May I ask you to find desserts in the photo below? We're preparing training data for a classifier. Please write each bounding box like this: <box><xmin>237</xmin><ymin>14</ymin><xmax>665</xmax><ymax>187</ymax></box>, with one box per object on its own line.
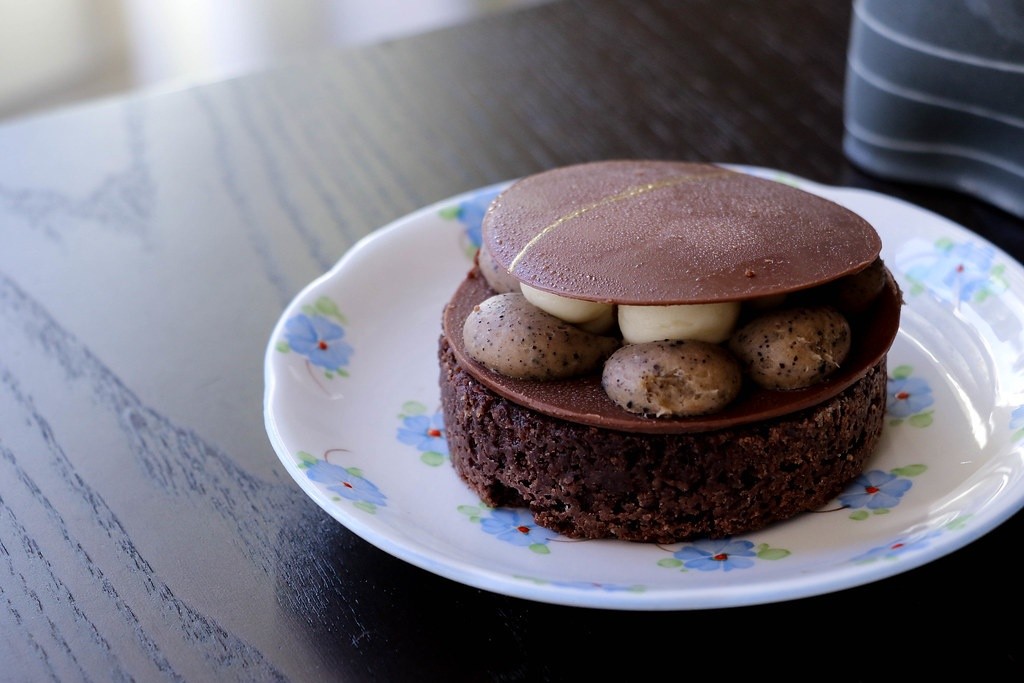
<box><xmin>437</xmin><ymin>160</ymin><xmax>904</xmax><ymax>540</ymax></box>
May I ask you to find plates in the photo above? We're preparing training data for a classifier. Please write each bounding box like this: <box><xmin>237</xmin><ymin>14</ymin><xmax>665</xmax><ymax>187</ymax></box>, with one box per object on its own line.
<box><xmin>264</xmin><ymin>163</ymin><xmax>1024</xmax><ymax>609</ymax></box>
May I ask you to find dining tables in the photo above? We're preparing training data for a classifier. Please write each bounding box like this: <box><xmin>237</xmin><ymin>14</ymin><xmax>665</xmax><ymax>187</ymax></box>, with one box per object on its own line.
<box><xmin>0</xmin><ymin>0</ymin><xmax>1024</xmax><ymax>683</ymax></box>
